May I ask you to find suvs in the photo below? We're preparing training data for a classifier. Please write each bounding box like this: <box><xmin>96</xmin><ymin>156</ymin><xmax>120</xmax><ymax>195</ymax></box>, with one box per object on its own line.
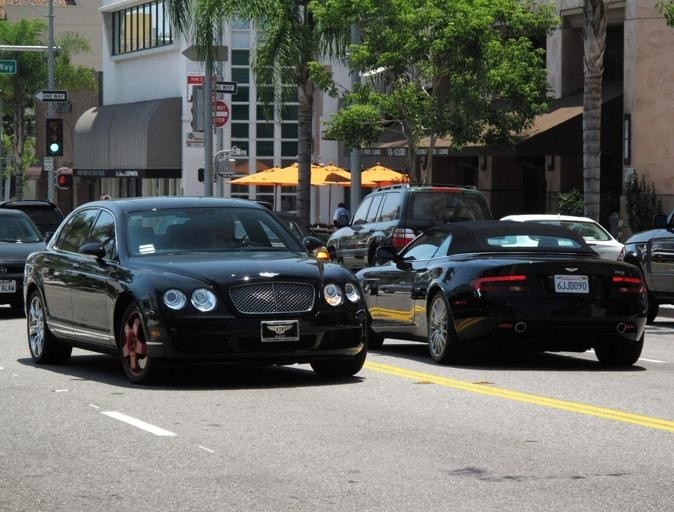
<box><xmin>325</xmin><ymin>184</ymin><xmax>494</xmax><ymax>272</ymax></box>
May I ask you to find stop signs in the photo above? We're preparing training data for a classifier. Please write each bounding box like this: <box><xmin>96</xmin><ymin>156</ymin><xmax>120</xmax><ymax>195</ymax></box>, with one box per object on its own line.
<box><xmin>212</xmin><ymin>100</ymin><xmax>229</xmax><ymax>128</ymax></box>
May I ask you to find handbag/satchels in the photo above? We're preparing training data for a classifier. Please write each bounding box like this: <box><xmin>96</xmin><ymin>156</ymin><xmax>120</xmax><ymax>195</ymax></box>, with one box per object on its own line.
<box><xmin>338</xmin><ymin>215</ymin><xmax>348</xmax><ymax>226</ymax></box>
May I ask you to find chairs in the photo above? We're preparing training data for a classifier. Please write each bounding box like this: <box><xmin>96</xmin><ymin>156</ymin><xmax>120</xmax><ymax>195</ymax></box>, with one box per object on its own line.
<box><xmin>166</xmin><ymin>223</ymin><xmax>199</xmax><ymax>250</ymax></box>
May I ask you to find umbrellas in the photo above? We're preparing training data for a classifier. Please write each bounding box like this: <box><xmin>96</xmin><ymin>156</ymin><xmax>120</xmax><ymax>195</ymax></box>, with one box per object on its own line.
<box><xmin>222</xmin><ymin>159</ymin><xmax>411</xmax><ymax>232</ymax></box>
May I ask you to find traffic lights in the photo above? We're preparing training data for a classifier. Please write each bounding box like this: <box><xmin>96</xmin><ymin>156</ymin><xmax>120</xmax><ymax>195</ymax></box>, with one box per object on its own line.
<box><xmin>57</xmin><ymin>174</ymin><xmax>73</xmax><ymax>188</ymax></box>
<box><xmin>46</xmin><ymin>118</ymin><xmax>63</xmax><ymax>156</ymax></box>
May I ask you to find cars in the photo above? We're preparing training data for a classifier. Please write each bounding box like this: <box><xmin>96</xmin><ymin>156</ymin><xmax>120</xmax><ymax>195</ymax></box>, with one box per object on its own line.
<box><xmin>353</xmin><ymin>219</ymin><xmax>649</xmax><ymax>368</ymax></box>
<box><xmin>498</xmin><ymin>213</ymin><xmax>627</xmax><ymax>262</ymax></box>
<box><xmin>1</xmin><ymin>199</ymin><xmax>65</xmax><ymax>241</ymax></box>
<box><xmin>0</xmin><ymin>208</ymin><xmax>54</xmax><ymax>317</ymax></box>
<box><xmin>624</xmin><ymin>210</ymin><xmax>674</xmax><ymax>325</ymax></box>
<box><xmin>23</xmin><ymin>195</ymin><xmax>371</xmax><ymax>384</ymax></box>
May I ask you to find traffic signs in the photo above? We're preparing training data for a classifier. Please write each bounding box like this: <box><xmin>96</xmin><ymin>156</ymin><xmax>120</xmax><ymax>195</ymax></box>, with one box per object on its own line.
<box><xmin>0</xmin><ymin>59</ymin><xmax>17</xmax><ymax>74</ymax></box>
<box><xmin>32</xmin><ymin>90</ymin><xmax>68</xmax><ymax>104</ymax></box>
<box><xmin>214</xmin><ymin>81</ymin><xmax>237</xmax><ymax>94</ymax></box>
